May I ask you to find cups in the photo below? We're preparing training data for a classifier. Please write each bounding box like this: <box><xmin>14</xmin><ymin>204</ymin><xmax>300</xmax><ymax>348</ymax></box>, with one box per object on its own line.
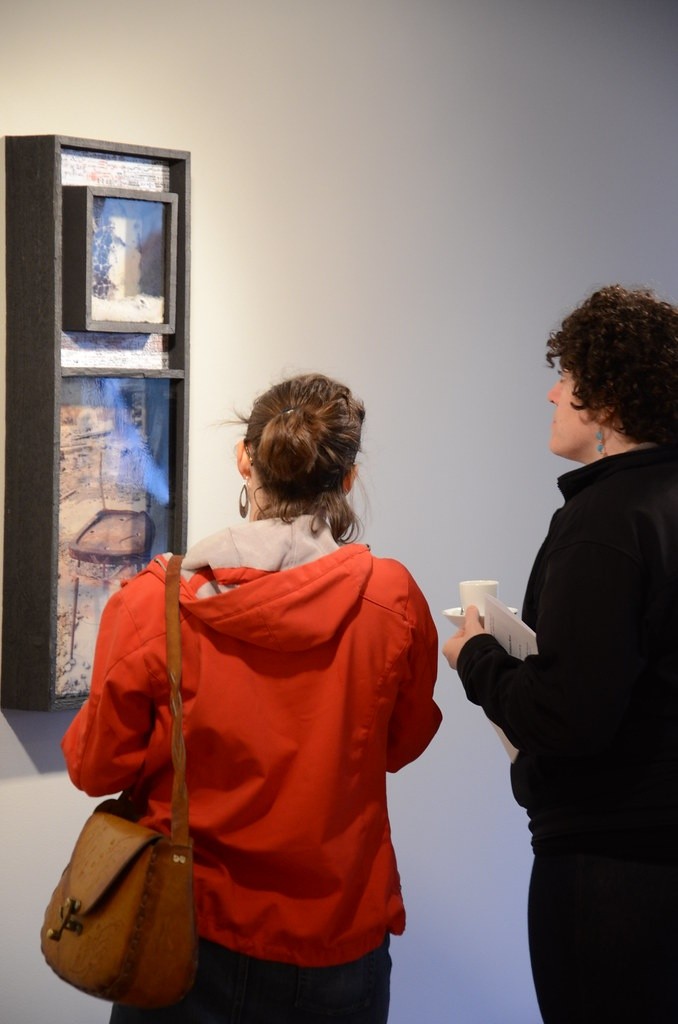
<box><xmin>459</xmin><ymin>580</ymin><xmax>499</xmax><ymax>617</ymax></box>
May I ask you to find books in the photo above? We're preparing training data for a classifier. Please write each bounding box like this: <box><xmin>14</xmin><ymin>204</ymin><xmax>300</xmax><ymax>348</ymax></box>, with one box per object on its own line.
<box><xmin>485</xmin><ymin>593</ymin><xmax>538</xmax><ymax>764</ymax></box>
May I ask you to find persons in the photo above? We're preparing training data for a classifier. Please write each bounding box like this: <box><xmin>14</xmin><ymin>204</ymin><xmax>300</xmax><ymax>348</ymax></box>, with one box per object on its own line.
<box><xmin>441</xmin><ymin>286</ymin><xmax>678</xmax><ymax>1023</ymax></box>
<box><xmin>60</xmin><ymin>373</ymin><xmax>443</xmax><ymax>1024</ymax></box>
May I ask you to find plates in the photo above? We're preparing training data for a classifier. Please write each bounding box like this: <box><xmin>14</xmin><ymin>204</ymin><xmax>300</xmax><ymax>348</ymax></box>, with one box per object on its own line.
<box><xmin>441</xmin><ymin>607</ymin><xmax>519</xmax><ymax>630</ymax></box>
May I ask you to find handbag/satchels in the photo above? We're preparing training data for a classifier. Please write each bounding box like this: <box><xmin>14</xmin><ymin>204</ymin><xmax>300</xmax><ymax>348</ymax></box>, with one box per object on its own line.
<box><xmin>40</xmin><ymin>798</ymin><xmax>197</xmax><ymax>1006</ymax></box>
